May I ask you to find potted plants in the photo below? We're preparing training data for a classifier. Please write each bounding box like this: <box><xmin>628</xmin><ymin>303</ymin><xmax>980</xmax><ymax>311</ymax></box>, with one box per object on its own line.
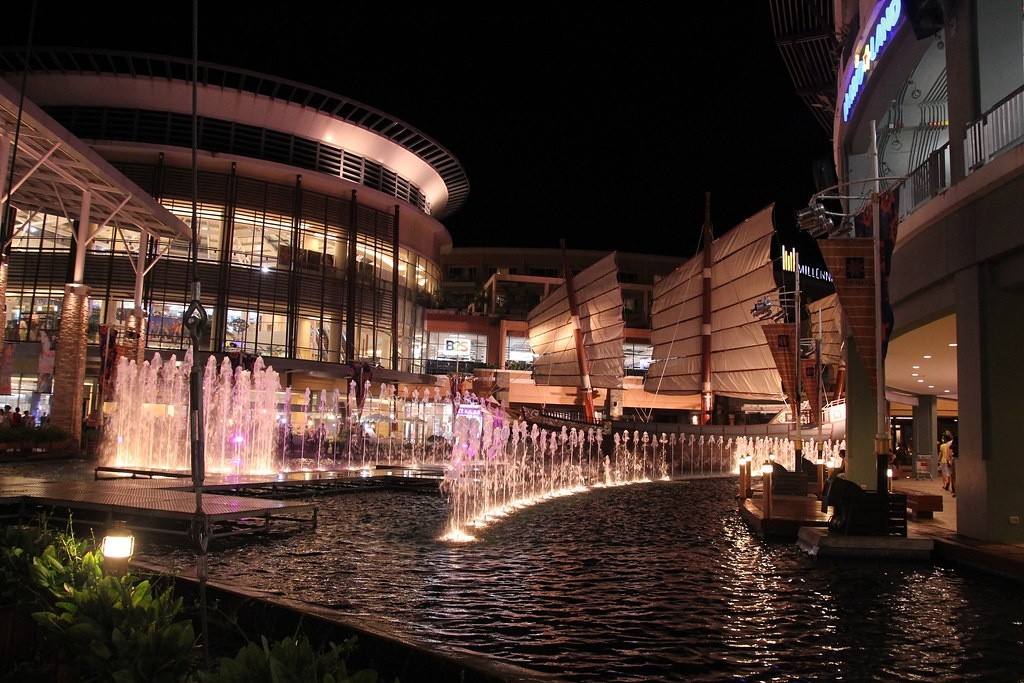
<box><xmin>0</xmin><ymin>423</ymin><xmax>72</xmax><ymax>451</ymax></box>
<box><xmin>83</xmin><ymin>429</ymin><xmax>97</xmax><ymax>449</ymax></box>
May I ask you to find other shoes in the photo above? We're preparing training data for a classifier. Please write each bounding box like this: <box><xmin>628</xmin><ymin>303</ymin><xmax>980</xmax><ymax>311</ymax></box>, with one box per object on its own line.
<box><xmin>943</xmin><ymin>482</ymin><xmax>949</xmax><ymax>490</ymax></box>
<box><xmin>951</xmin><ymin>490</ymin><xmax>955</xmax><ymax>493</ymax></box>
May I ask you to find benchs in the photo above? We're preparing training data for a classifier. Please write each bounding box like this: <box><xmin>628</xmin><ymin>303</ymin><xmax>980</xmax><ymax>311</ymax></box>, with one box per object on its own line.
<box><xmin>891</xmin><ymin>486</ymin><xmax>944</xmax><ymax>521</ymax></box>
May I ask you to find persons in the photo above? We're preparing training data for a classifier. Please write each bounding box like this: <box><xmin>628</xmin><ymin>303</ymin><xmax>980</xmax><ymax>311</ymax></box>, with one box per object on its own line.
<box><xmin>817</xmin><ymin>449</ymin><xmax>845</xmax><ymax>501</ymax></box>
<box><xmin>889</xmin><ymin>451</ymin><xmax>899</xmax><ymax>477</ymax></box>
<box><xmin>38</xmin><ymin>373</ymin><xmax>51</xmax><ymax>392</ymax></box>
<box><xmin>935</xmin><ymin>431</ymin><xmax>958</xmax><ymax>497</ymax></box>
<box><xmin>0</xmin><ymin>406</ymin><xmax>48</xmax><ymax>431</ymax></box>
